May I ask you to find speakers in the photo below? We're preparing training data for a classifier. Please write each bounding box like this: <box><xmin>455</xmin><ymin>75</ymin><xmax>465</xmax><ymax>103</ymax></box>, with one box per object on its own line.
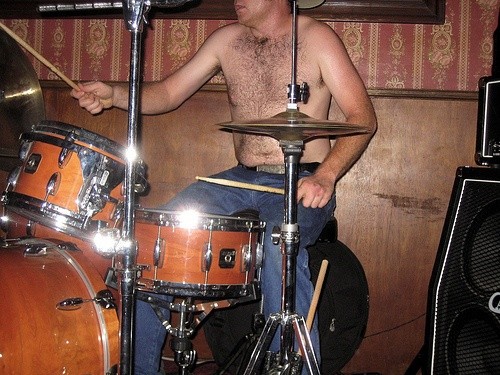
<box><xmin>422</xmin><ymin>167</ymin><xmax>500</xmax><ymax>374</ymax></box>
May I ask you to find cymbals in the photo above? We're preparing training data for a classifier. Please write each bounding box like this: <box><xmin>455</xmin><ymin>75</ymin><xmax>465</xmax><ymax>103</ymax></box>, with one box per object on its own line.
<box><xmin>215</xmin><ymin>111</ymin><xmax>372</xmax><ymax>140</ymax></box>
<box><xmin>0</xmin><ymin>31</ymin><xmax>46</xmax><ymax>170</ymax></box>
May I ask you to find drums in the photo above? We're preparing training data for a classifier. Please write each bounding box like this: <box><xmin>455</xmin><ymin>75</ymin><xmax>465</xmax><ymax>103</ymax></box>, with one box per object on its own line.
<box><xmin>104</xmin><ymin>205</ymin><xmax>267</xmax><ymax>297</ymax></box>
<box><xmin>0</xmin><ymin>236</ymin><xmax>122</xmax><ymax>374</ymax></box>
<box><xmin>2</xmin><ymin>120</ymin><xmax>149</xmax><ymax>258</ymax></box>
